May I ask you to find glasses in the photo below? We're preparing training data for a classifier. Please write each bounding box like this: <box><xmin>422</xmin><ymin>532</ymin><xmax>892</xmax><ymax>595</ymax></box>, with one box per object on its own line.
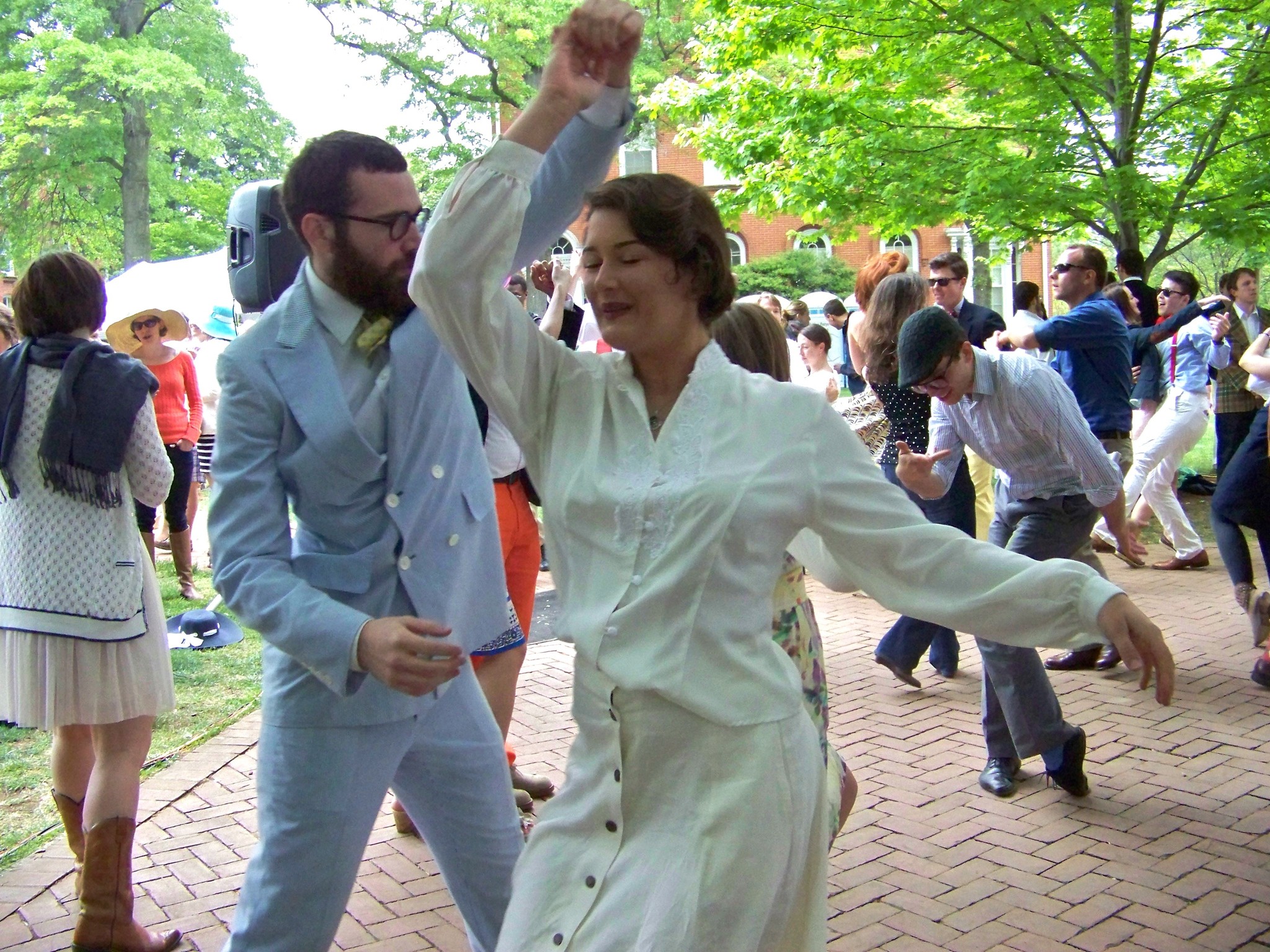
<box><xmin>132</xmin><ymin>319</ymin><xmax>159</xmax><ymax>331</ymax></box>
<box><xmin>1156</xmin><ymin>288</ymin><xmax>1185</xmax><ymax>297</ymax></box>
<box><xmin>339</xmin><ymin>209</ymin><xmax>430</xmax><ymax>241</ymax></box>
<box><xmin>928</xmin><ymin>277</ymin><xmax>961</xmax><ymax>287</ymax></box>
<box><xmin>1114</xmin><ymin>265</ymin><xmax>1119</xmax><ymax>271</ymax></box>
<box><xmin>1051</xmin><ymin>264</ymin><xmax>1088</xmax><ymax>273</ymax></box>
<box><xmin>911</xmin><ymin>348</ymin><xmax>956</xmax><ymax>394</ymax></box>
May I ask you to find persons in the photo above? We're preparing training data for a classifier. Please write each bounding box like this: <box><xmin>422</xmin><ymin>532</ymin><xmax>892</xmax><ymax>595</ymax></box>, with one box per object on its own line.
<box><xmin>408</xmin><ymin>11</ymin><xmax>1175</xmax><ymax>952</ymax></box>
<box><xmin>205</xmin><ymin>1</ymin><xmax>646</xmax><ymax>952</ymax></box>
<box><xmin>394</xmin><ymin>255</ymin><xmax>616</xmax><ymax>835</ymax></box>
<box><xmin>0</xmin><ymin>251</ymin><xmax>185</xmax><ymax>952</ymax></box>
<box><xmin>707</xmin><ymin>302</ymin><xmax>856</xmax><ymax>852</ymax></box>
<box><xmin>895</xmin><ymin>306</ymin><xmax>1153</xmax><ymax>798</ymax></box>
<box><xmin>0</xmin><ymin>300</ymin><xmax>243</xmax><ymax>601</ymax></box>
<box><xmin>740</xmin><ymin>244</ymin><xmax>1270</xmax><ymax>682</ymax></box>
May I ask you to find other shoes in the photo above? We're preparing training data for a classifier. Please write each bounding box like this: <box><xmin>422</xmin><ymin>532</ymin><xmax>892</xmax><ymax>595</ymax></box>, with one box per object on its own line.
<box><xmin>1251</xmin><ymin>648</ymin><xmax>1270</xmax><ymax>687</ymax></box>
<box><xmin>1089</xmin><ymin>530</ymin><xmax>1210</xmax><ymax>569</ymax></box>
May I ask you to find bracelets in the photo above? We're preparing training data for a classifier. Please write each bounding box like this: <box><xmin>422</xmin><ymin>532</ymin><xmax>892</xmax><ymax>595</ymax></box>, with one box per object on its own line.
<box><xmin>1262</xmin><ymin>333</ymin><xmax>1269</xmax><ymax>336</ymax></box>
<box><xmin>1212</xmin><ymin>338</ymin><xmax>1222</xmax><ymax>345</ymax></box>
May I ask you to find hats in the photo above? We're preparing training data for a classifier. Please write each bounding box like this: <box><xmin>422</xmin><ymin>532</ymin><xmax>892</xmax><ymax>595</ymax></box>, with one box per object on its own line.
<box><xmin>898</xmin><ymin>306</ymin><xmax>966</xmax><ymax>390</ymax></box>
<box><xmin>168</xmin><ymin>609</ymin><xmax>243</xmax><ymax>649</ymax></box>
<box><xmin>105</xmin><ymin>309</ymin><xmax>188</xmax><ymax>352</ymax></box>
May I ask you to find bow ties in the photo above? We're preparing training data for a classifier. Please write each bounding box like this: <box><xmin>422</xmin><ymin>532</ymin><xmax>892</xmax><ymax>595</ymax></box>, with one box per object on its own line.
<box><xmin>949</xmin><ymin>311</ymin><xmax>960</xmax><ymax>319</ymax></box>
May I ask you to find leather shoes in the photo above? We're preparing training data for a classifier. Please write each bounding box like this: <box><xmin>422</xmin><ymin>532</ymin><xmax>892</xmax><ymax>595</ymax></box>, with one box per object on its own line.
<box><xmin>978</xmin><ymin>757</ymin><xmax>1021</xmax><ymax>796</ymax></box>
<box><xmin>1042</xmin><ymin>728</ymin><xmax>1089</xmax><ymax>797</ymax></box>
<box><xmin>875</xmin><ymin>654</ymin><xmax>921</xmax><ymax>688</ymax></box>
<box><xmin>1045</xmin><ymin>647</ymin><xmax>1097</xmax><ymax>671</ymax></box>
<box><xmin>1096</xmin><ymin>645</ymin><xmax>1121</xmax><ymax>670</ymax></box>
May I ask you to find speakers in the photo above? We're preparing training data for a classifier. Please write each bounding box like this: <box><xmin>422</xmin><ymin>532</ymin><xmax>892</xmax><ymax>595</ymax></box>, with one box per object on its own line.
<box><xmin>226</xmin><ymin>178</ymin><xmax>305</xmax><ymax>314</ymax></box>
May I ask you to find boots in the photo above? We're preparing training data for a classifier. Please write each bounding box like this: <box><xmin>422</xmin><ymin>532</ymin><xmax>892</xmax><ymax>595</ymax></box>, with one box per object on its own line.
<box><xmin>170</xmin><ymin>525</ymin><xmax>204</xmax><ymax>600</ymax></box>
<box><xmin>51</xmin><ymin>789</ymin><xmax>84</xmax><ymax>898</ymax></box>
<box><xmin>72</xmin><ymin>816</ymin><xmax>182</xmax><ymax>952</ymax></box>
<box><xmin>1234</xmin><ymin>582</ymin><xmax>1270</xmax><ymax>647</ymax></box>
<box><xmin>141</xmin><ymin>532</ymin><xmax>155</xmax><ymax>569</ymax></box>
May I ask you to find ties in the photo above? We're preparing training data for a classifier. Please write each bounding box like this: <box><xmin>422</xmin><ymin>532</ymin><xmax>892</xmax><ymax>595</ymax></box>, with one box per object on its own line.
<box><xmin>356</xmin><ymin>316</ymin><xmax>394</xmax><ymax>356</ymax></box>
<box><xmin>1169</xmin><ymin>331</ymin><xmax>1178</xmax><ymax>383</ymax></box>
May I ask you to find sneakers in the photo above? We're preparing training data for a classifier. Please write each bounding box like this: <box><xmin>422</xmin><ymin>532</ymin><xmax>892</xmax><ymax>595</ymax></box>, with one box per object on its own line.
<box><xmin>156</xmin><ymin>538</ymin><xmax>193</xmax><ymax>552</ymax></box>
<box><xmin>394</xmin><ymin>787</ymin><xmax>533</xmax><ymax>834</ymax></box>
<box><xmin>507</xmin><ymin>755</ymin><xmax>554</xmax><ymax>797</ymax></box>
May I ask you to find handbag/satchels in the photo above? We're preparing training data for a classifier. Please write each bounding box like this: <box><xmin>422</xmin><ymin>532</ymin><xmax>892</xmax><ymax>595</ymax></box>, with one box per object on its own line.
<box><xmin>1181</xmin><ymin>475</ymin><xmax>1218</xmax><ymax>495</ymax></box>
